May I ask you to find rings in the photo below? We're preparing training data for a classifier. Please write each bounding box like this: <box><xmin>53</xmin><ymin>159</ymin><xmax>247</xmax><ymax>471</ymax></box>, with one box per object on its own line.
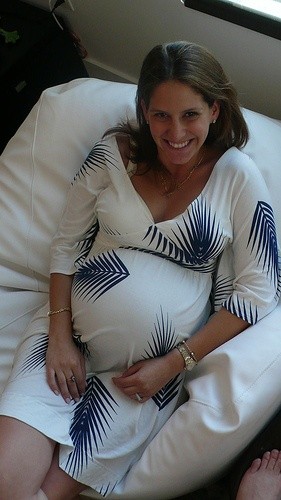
<box><xmin>67</xmin><ymin>376</ymin><xmax>76</xmax><ymax>382</ymax></box>
<box><xmin>134</xmin><ymin>393</ymin><xmax>143</xmax><ymax>402</ymax></box>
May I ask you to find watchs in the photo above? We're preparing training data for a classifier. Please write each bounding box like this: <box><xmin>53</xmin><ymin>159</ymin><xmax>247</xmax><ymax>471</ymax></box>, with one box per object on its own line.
<box><xmin>176</xmin><ymin>344</ymin><xmax>198</xmax><ymax>371</ymax></box>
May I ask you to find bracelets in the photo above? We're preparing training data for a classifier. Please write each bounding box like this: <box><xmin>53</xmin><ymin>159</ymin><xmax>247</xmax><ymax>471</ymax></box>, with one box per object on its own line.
<box><xmin>47</xmin><ymin>307</ymin><xmax>71</xmax><ymax>316</ymax></box>
<box><xmin>183</xmin><ymin>340</ymin><xmax>198</xmax><ymax>363</ymax></box>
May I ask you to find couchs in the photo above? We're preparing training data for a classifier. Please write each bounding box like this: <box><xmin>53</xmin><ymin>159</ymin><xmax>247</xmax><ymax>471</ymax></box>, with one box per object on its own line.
<box><xmin>0</xmin><ymin>78</ymin><xmax>280</xmax><ymax>499</ymax></box>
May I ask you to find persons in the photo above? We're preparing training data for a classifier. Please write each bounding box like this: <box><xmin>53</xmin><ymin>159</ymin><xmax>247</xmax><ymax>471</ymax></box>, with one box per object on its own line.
<box><xmin>0</xmin><ymin>42</ymin><xmax>281</xmax><ymax>500</ymax></box>
<box><xmin>237</xmin><ymin>449</ymin><xmax>281</xmax><ymax>500</ymax></box>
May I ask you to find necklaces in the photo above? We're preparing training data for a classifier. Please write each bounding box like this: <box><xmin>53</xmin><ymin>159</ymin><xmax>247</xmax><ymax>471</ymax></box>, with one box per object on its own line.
<box><xmin>160</xmin><ymin>150</ymin><xmax>205</xmax><ymax>199</ymax></box>
